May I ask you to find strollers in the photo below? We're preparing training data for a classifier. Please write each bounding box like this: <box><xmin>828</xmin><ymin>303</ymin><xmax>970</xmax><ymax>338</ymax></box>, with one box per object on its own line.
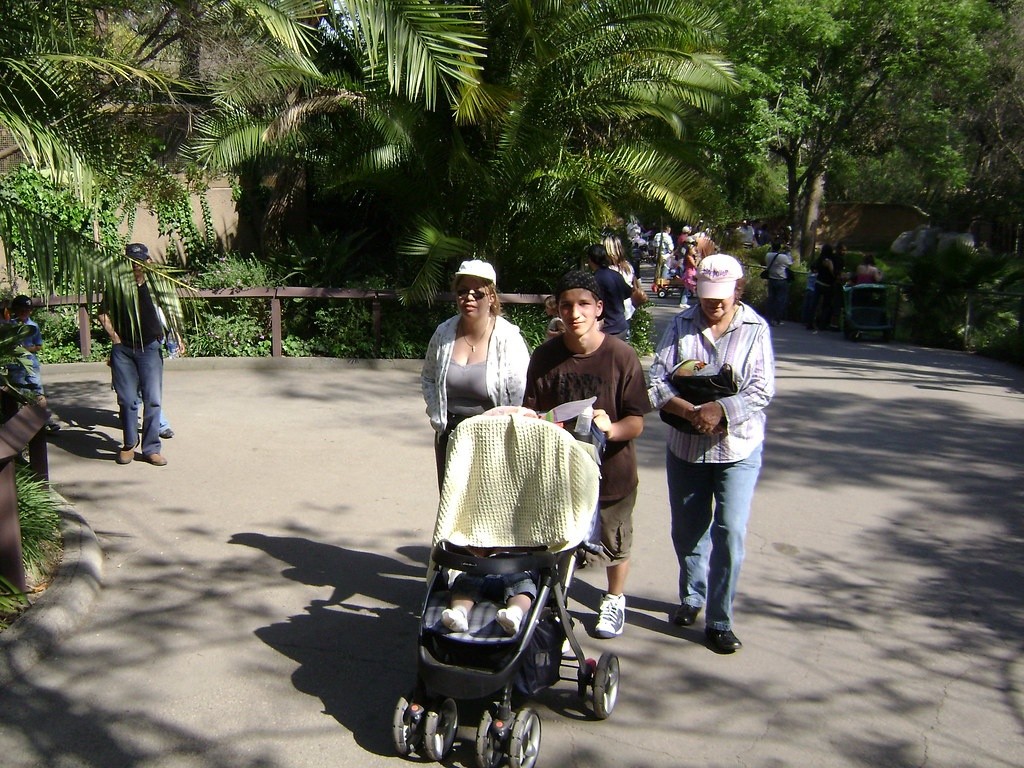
<box><xmin>392</xmin><ymin>412</ymin><xmax>621</xmax><ymax>768</ymax></box>
<box><xmin>843</xmin><ymin>282</ymin><xmax>894</xmax><ymax>341</ymax></box>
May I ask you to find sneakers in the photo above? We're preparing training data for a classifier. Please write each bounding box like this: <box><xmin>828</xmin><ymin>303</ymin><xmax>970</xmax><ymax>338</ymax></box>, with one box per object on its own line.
<box><xmin>594</xmin><ymin>592</ymin><xmax>626</xmax><ymax>638</ymax></box>
<box><xmin>44</xmin><ymin>421</ymin><xmax>60</xmax><ymax>431</ymax></box>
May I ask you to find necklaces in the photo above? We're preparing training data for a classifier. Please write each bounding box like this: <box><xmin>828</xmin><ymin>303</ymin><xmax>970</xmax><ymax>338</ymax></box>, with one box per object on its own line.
<box><xmin>461</xmin><ymin>318</ymin><xmax>491</xmax><ymax>352</ymax></box>
<box><xmin>128</xmin><ymin>274</ymin><xmax>157</xmax><ymax>294</ymax></box>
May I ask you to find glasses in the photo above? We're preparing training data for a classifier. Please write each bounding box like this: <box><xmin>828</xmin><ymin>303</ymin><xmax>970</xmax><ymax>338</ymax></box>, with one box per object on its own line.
<box><xmin>457</xmin><ymin>288</ymin><xmax>489</xmax><ymax>300</ymax></box>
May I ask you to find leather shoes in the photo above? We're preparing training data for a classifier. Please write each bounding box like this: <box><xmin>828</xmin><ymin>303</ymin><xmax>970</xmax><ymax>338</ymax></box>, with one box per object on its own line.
<box><xmin>673</xmin><ymin>601</ymin><xmax>701</xmax><ymax>625</ymax></box>
<box><xmin>705</xmin><ymin>625</ymin><xmax>742</xmax><ymax>649</ymax></box>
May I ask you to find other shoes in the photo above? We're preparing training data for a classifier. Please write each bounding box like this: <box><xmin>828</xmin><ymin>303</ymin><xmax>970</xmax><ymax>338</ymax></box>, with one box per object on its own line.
<box><xmin>768</xmin><ymin>319</ymin><xmax>785</xmax><ymax>325</ymax></box>
<box><xmin>441</xmin><ymin>609</ymin><xmax>469</xmax><ymax>632</ymax></box>
<box><xmin>161</xmin><ymin>429</ymin><xmax>174</xmax><ymax>438</ymax></box>
<box><xmin>495</xmin><ymin>608</ymin><xmax>520</xmax><ymax>634</ymax></box>
<box><xmin>116</xmin><ymin>434</ymin><xmax>140</xmax><ymax>463</ymax></box>
<box><xmin>679</xmin><ymin>303</ymin><xmax>690</xmax><ymax>308</ymax></box>
<box><xmin>143</xmin><ymin>451</ymin><xmax>167</xmax><ymax>466</ymax></box>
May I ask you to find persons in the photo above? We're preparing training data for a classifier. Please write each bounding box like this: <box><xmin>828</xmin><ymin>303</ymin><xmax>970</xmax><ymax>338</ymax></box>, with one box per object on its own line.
<box><xmin>649</xmin><ymin>254</ymin><xmax>776</xmax><ymax>651</ymax></box>
<box><xmin>765</xmin><ymin>237</ymin><xmax>882</xmax><ymax>331</ymax></box>
<box><xmin>544</xmin><ymin>294</ymin><xmax>568</xmax><ymax>348</ymax></box>
<box><xmin>652</xmin><ymin>225</ymin><xmax>715</xmax><ymax>309</ymax></box>
<box><xmin>98</xmin><ymin>243</ymin><xmax>185</xmax><ymax>466</ymax></box>
<box><xmin>734</xmin><ymin>218</ymin><xmax>767</xmax><ymax>250</ymax></box>
<box><xmin>521</xmin><ymin>270</ymin><xmax>652</xmax><ymax>640</ymax></box>
<box><xmin>441</xmin><ymin>546</ymin><xmax>539</xmax><ymax>636</ymax></box>
<box><xmin>0</xmin><ymin>295</ymin><xmax>47</xmax><ymax>409</ymax></box>
<box><xmin>421</xmin><ymin>260</ymin><xmax>532</xmax><ymax>498</ymax></box>
<box><xmin>587</xmin><ymin>234</ymin><xmax>636</xmax><ymax>342</ymax></box>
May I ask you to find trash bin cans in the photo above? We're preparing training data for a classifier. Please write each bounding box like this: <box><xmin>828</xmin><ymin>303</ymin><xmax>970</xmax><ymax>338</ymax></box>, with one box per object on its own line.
<box><xmin>0</xmin><ymin>386</ymin><xmax>53</xmax><ymax>593</ymax></box>
<box><xmin>841</xmin><ymin>283</ymin><xmax>894</xmax><ymax>341</ymax></box>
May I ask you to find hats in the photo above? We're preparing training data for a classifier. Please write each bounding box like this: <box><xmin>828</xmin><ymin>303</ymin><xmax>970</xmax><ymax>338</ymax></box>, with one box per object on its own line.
<box><xmin>12</xmin><ymin>294</ymin><xmax>33</xmax><ymax>310</ymax></box>
<box><xmin>125</xmin><ymin>243</ymin><xmax>148</xmax><ymax>262</ymax></box>
<box><xmin>695</xmin><ymin>253</ymin><xmax>744</xmax><ymax>299</ymax></box>
<box><xmin>682</xmin><ymin>226</ymin><xmax>692</xmax><ymax>234</ymax></box>
<box><xmin>455</xmin><ymin>260</ymin><xmax>497</xmax><ymax>288</ymax></box>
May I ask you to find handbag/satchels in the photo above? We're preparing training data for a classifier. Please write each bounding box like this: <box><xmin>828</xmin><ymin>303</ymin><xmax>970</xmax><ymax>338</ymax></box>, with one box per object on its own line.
<box><xmin>660</xmin><ymin>358</ymin><xmax>737</xmax><ymax>436</ymax></box>
<box><xmin>760</xmin><ymin>270</ymin><xmax>771</xmax><ymax>280</ymax></box>
<box><xmin>632</xmin><ymin>280</ymin><xmax>649</xmax><ymax>306</ymax></box>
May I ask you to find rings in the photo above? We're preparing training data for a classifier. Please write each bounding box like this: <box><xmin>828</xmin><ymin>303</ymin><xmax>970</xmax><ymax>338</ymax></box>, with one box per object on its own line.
<box><xmin>696</xmin><ymin>424</ymin><xmax>702</xmax><ymax>429</ymax></box>
<box><xmin>693</xmin><ymin>405</ymin><xmax>702</xmax><ymax>411</ymax></box>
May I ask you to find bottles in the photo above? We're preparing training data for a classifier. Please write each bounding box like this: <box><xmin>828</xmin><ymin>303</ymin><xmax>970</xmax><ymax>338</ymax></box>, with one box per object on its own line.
<box><xmin>167</xmin><ymin>341</ymin><xmax>179</xmax><ymax>360</ymax></box>
<box><xmin>574</xmin><ymin>404</ymin><xmax>593</xmax><ymax>435</ymax></box>
